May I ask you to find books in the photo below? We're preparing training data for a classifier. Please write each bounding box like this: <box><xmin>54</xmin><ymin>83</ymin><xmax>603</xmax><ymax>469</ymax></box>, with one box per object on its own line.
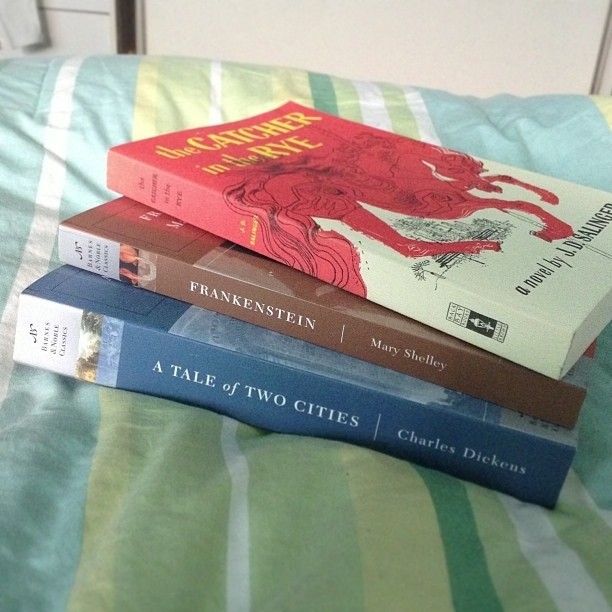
<box><xmin>56</xmin><ymin>194</ymin><xmax>596</xmax><ymax>428</ymax></box>
<box><xmin>14</xmin><ymin>265</ymin><xmax>578</xmax><ymax>511</ymax></box>
<box><xmin>106</xmin><ymin>101</ymin><xmax>611</xmax><ymax>380</ymax></box>
